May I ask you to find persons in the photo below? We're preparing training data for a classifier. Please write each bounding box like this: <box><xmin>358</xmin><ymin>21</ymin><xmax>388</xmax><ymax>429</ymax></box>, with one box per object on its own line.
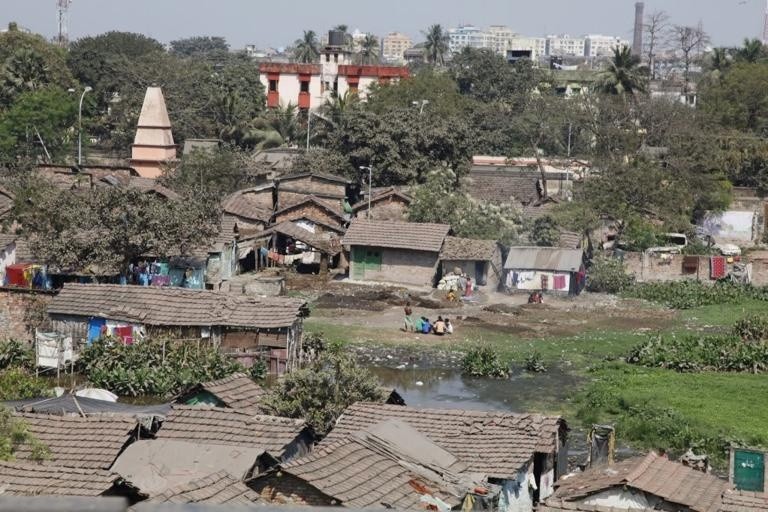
<box><xmin>416</xmin><ymin>314</ymin><xmax>454</xmax><ymax>335</ymax></box>
<box><xmin>529</xmin><ymin>291</ymin><xmax>544</xmax><ymax>304</ymax></box>
<box><xmin>288</xmin><ymin>240</ymin><xmax>295</xmax><ymax>255</ymax></box>
<box><xmin>657</xmin><ymin>446</ymin><xmax>669</xmax><ymax>459</ymax></box>
<box><xmin>465</xmin><ymin>278</ymin><xmax>472</xmax><ymax>297</ymax></box>
<box><xmin>403</xmin><ymin>301</ymin><xmax>414</xmax><ymax>332</ymax></box>
<box><xmin>447</xmin><ymin>289</ymin><xmax>456</xmax><ymax>301</ymax></box>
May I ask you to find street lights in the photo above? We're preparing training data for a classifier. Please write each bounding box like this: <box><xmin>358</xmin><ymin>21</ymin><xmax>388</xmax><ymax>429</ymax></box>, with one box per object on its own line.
<box><xmin>419</xmin><ymin>99</ymin><xmax>431</xmax><ymax>116</ymax></box>
<box><xmin>359</xmin><ymin>163</ymin><xmax>372</xmax><ymax>220</ymax></box>
<box><xmin>77</xmin><ymin>84</ymin><xmax>93</xmax><ymax>164</ymax></box>
<box><xmin>67</xmin><ymin>88</ymin><xmax>76</xmax><ymax>98</ymax></box>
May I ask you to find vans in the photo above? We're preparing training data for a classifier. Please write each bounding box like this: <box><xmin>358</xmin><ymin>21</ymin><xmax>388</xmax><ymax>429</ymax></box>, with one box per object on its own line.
<box><xmin>650</xmin><ymin>232</ymin><xmax>688</xmax><ymax>256</ymax></box>
